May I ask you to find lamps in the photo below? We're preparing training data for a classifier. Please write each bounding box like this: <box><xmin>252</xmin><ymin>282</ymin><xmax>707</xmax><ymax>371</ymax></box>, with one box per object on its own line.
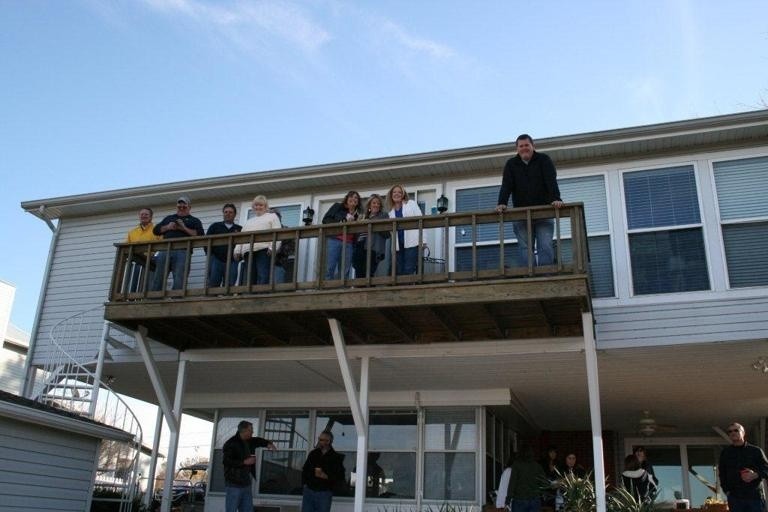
<box><xmin>433</xmin><ymin>193</ymin><xmax>449</xmax><ymax>214</ymax></box>
<box><xmin>637</xmin><ymin>426</ymin><xmax>655</xmax><ymax>437</ymax></box>
<box><xmin>301</xmin><ymin>205</ymin><xmax>315</xmax><ymax>226</ymax></box>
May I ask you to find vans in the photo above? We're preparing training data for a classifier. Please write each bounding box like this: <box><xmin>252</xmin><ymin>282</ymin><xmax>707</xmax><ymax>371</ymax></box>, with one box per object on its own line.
<box><xmin>155</xmin><ymin>462</ymin><xmax>209</xmax><ymax>505</ymax></box>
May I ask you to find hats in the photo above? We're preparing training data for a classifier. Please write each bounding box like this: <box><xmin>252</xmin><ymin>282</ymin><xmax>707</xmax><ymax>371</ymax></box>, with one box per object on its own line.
<box><xmin>177</xmin><ymin>196</ymin><xmax>190</xmax><ymax>205</ymax></box>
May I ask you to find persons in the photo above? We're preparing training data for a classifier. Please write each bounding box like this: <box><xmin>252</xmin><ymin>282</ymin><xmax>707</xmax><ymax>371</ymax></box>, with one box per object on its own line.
<box><xmin>204</xmin><ymin>204</ymin><xmax>243</xmax><ymax>297</ymax></box>
<box><xmin>222</xmin><ymin>422</ymin><xmax>276</xmax><ymax>512</ymax></box>
<box><xmin>321</xmin><ymin>190</ymin><xmax>361</xmax><ymax>290</ymax></box>
<box><xmin>301</xmin><ymin>429</ymin><xmax>347</xmax><ymax>512</ymax></box>
<box><xmin>351</xmin><ymin>194</ymin><xmax>392</xmax><ymax>288</ymax></box>
<box><xmin>718</xmin><ymin>421</ymin><xmax>767</xmax><ymax>511</ymax></box>
<box><xmin>494</xmin><ymin>134</ymin><xmax>564</xmax><ymax>278</ymax></box>
<box><xmin>385</xmin><ymin>184</ymin><xmax>428</xmax><ymax>286</ymax></box>
<box><xmin>267</xmin><ymin>208</ymin><xmax>293</xmax><ymax>283</ymax></box>
<box><xmin>233</xmin><ymin>195</ymin><xmax>281</xmax><ymax>294</ymax></box>
<box><xmin>490</xmin><ymin>443</ymin><xmax>660</xmax><ymax>510</ymax></box>
<box><xmin>150</xmin><ymin>196</ymin><xmax>205</xmax><ymax>300</ymax></box>
<box><xmin>123</xmin><ymin>207</ymin><xmax>161</xmax><ymax>302</ymax></box>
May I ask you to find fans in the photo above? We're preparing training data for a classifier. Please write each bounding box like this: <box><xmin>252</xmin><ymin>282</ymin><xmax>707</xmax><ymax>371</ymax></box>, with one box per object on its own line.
<box><xmin>611</xmin><ymin>408</ymin><xmax>676</xmax><ymax>428</ymax></box>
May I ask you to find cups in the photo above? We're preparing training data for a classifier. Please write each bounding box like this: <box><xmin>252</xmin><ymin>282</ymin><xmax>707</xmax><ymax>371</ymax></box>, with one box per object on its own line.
<box><xmin>315</xmin><ymin>467</ymin><xmax>321</xmax><ymax>476</ymax></box>
<box><xmin>251</xmin><ymin>454</ymin><xmax>255</xmax><ymax>457</ymax></box>
<box><xmin>740</xmin><ymin>470</ymin><xmax>751</xmax><ymax>482</ymax></box>
<box><xmin>346</xmin><ymin>214</ymin><xmax>354</xmax><ymax>222</ymax></box>
